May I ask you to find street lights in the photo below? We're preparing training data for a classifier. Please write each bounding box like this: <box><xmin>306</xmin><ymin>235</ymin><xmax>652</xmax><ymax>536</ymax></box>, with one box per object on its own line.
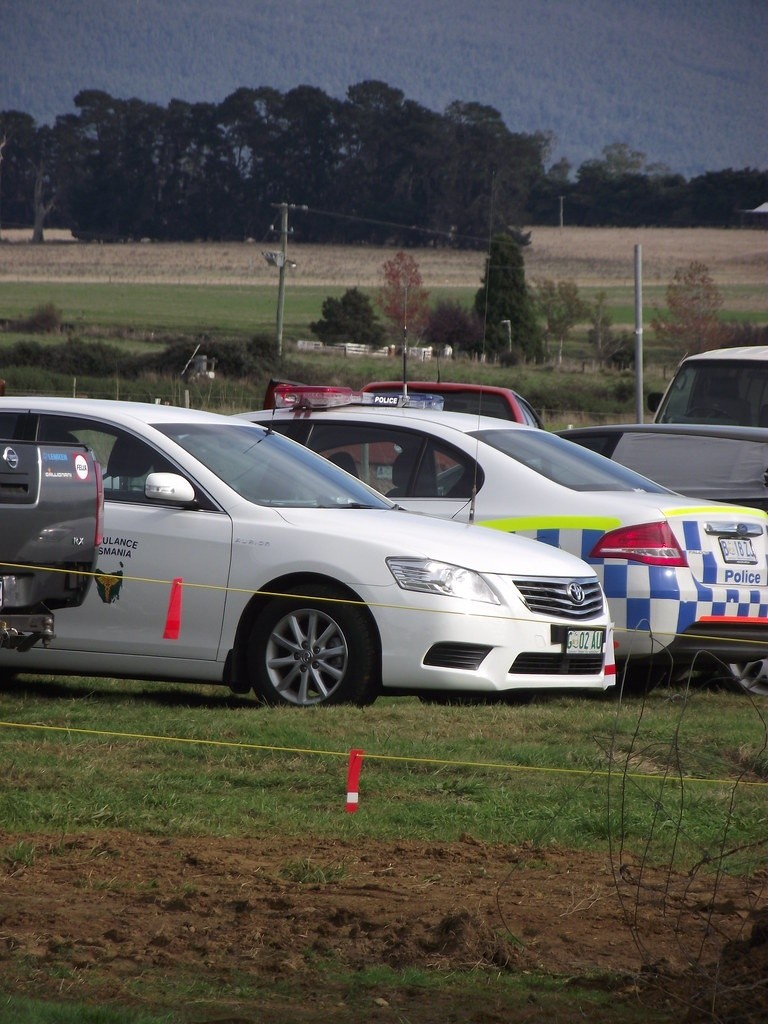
<box><xmin>501</xmin><ymin>319</ymin><xmax>512</xmax><ymax>353</ymax></box>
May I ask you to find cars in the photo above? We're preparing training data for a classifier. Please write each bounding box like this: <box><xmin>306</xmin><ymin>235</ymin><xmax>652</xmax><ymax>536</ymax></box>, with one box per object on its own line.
<box><xmin>0</xmin><ymin>395</ymin><xmax>619</xmax><ymax>712</ymax></box>
<box><xmin>537</xmin><ymin>346</ymin><xmax>768</xmax><ymax>517</ymax></box>
<box><xmin>262</xmin><ymin>380</ymin><xmax>546</xmax><ymax>497</ymax></box>
<box><xmin>102</xmin><ymin>385</ymin><xmax>768</xmax><ymax>700</ymax></box>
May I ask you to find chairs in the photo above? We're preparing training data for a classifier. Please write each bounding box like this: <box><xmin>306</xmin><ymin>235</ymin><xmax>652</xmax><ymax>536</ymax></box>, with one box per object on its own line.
<box><xmin>385</xmin><ymin>450</ymin><xmax>415</xmax><ymax>498</ymax></box>
<box><xmin>102</xmin><ymin>435</ymin><xmax>153</xmax><ymax>492</ymax></box>
<box><xmin>688</xmin><ymin>376</ymin><xmax>752</xmax><ymax>425</ymax></box>
<box><xmin>326</xmin><ymin>451</ymin><xmax>359</xmax><ymax>481</ymax></box>
